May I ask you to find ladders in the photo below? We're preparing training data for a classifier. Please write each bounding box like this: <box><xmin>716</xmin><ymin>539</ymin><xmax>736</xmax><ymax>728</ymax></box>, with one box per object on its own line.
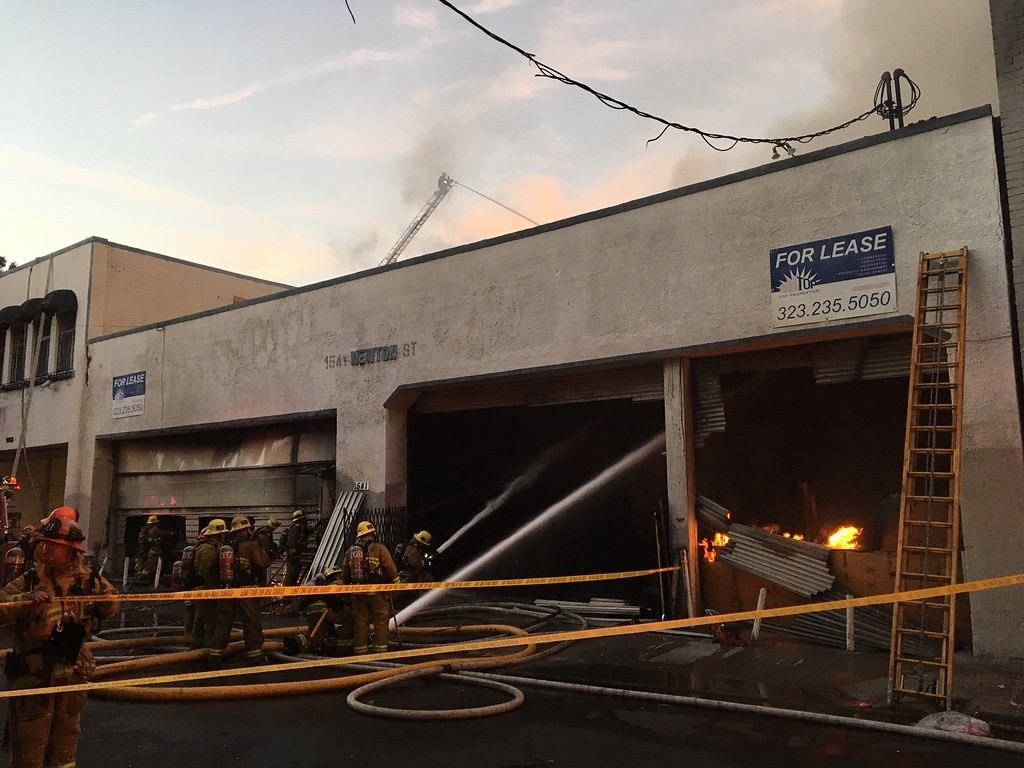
<box><xmin>887</xmin><ymin>244</ymin><xmax>971</xmax><ymax>713</ymax></box>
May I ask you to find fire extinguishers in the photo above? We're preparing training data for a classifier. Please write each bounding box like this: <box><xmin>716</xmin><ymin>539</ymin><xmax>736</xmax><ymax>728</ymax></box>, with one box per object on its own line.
<box><xmin>350</xmin><ymin>545</ymin><xmax>365</xmax><ymax>581</ymax></box>
<box><xmin>4</xmin><ymin>547</ymin><xmax>24</xmax><ymax>590</ymax></box>
<box><xmin>392</xmin><ymin>544</ymin><xmax>405</xmax><ymax>563</ymax></box>
<box><xmin>219</xmin><ymin>546</ymin><xmax>234</xmax><ymax>587</ymax></box>
<box><xmin>178</xmin><ymin>546</ymin><xmax>196</xmax><ymax>586</ymax></box>
<box><xmin>171</xmin><ymin>561</ymin><xmax>183</xmax><ymax>593</ymax></box>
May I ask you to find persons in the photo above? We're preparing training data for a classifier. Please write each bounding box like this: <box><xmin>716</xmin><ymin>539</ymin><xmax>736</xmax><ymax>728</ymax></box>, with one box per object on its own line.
<box><xmin>281</xmin><ymin>565</ymin><xmax>356</xmax><ymax>657</ymax></box>
<box><xmin>133</xmin><ymin>515</ymin><xmax>181</xmax><ymax>581</ymax></box>
<box><xmin>283</xmin><ymin>509</ymin><xmax>323</xmax><ymax>601</ymax></box>
<box><xmin>184</xmin><ymin>515</ymin><xmax>282</xmax><ymax>670</ymax></box>
<box><xmin>393</xmin><ymin>530</ymin><xmax>431</xmax><ymax>626</ymax></box>
<box><xmin>0</xmin><ymin>507</ymin><xmax>124</xmax><ymax>768</ymax></box>
<box><xmin>341</xmin><ymin>521</ymin><xmax>402</xmax><ymax>660</ymax></box>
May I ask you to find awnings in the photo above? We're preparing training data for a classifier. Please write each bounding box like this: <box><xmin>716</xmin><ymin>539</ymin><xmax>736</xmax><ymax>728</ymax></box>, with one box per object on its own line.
<box><xmin>16</xmin><ymin>298</ymin><xmax>42</xmax><ymax>323</ymax></box>
<box><xmin>0</xmin><ymin>305</ymin><xmax>26</xmax><ymax>324</ymax></box>
<box><xmin>37</xmin><ymin>290</ymin><xmax>78</xmax><ymax>313</ymax></box>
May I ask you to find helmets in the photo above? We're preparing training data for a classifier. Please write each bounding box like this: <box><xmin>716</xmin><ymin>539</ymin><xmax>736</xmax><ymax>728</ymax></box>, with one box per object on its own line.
<box><xmin>146</xmin><ymin>516</ymin><xmax>159</xmax><ymax>524</ymax></box>
<box><xmin>203</xmin><ymin>519</ymin><xmax>229</xmax><ymax>535</ymax></box>
<box><xmin>324</xmin><ymin>566</ymin><xmax>343</xmax><ymax>576</ymax></box>
<box><xmin>36</xmin><ymin>517</ymin><xmax>88</xmax><ymax>553</ymax></box>
<box><xmin>266</xmin><ymin>519</ymin><xmax>279</xmax><ymax>529</ymax></box>
<box><xmin>41</xmin><ymin>507</ymin><xmax>78</xmax><ymax>525</ymax></box>
<box><xmin>292</xmin><ymin>510</ymin><xmax>305</xmax><ymax>521</ymax></box>
<box><xmin>414</xmin><ymin>531</ymin><xmax>431</xmax><ymax>545</ymax></box>
<box><xmin>355</xmin><ymin>521</ymin><xmax>376</xmax><ymax>538</ymax></box>
<box><xmin>229</xmin><ymin>517</ymin><xmax>251</xmax><ymax>533</ymax></box>
